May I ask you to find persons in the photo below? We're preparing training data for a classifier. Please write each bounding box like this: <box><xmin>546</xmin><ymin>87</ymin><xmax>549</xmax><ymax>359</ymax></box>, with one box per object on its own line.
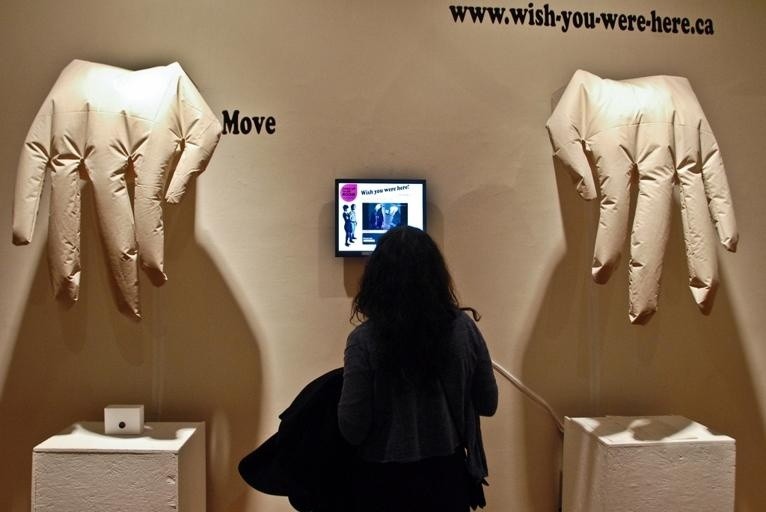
<box><xmin>338</xmin><ymin>225</ymin><xmax>498</xmax><ymax>512</ymax></box>
<box><xmin>342</xmin><ymin>204</ymin><xmax>357</xmax><ymax>246</ymax></box>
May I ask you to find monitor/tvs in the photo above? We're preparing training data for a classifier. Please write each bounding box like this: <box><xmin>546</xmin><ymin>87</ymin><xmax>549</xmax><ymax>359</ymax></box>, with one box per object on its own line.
<box><xmin>334</xmin><ymin>177</ymin><xmax>428</xmax><ymax>258</ymax></box>
